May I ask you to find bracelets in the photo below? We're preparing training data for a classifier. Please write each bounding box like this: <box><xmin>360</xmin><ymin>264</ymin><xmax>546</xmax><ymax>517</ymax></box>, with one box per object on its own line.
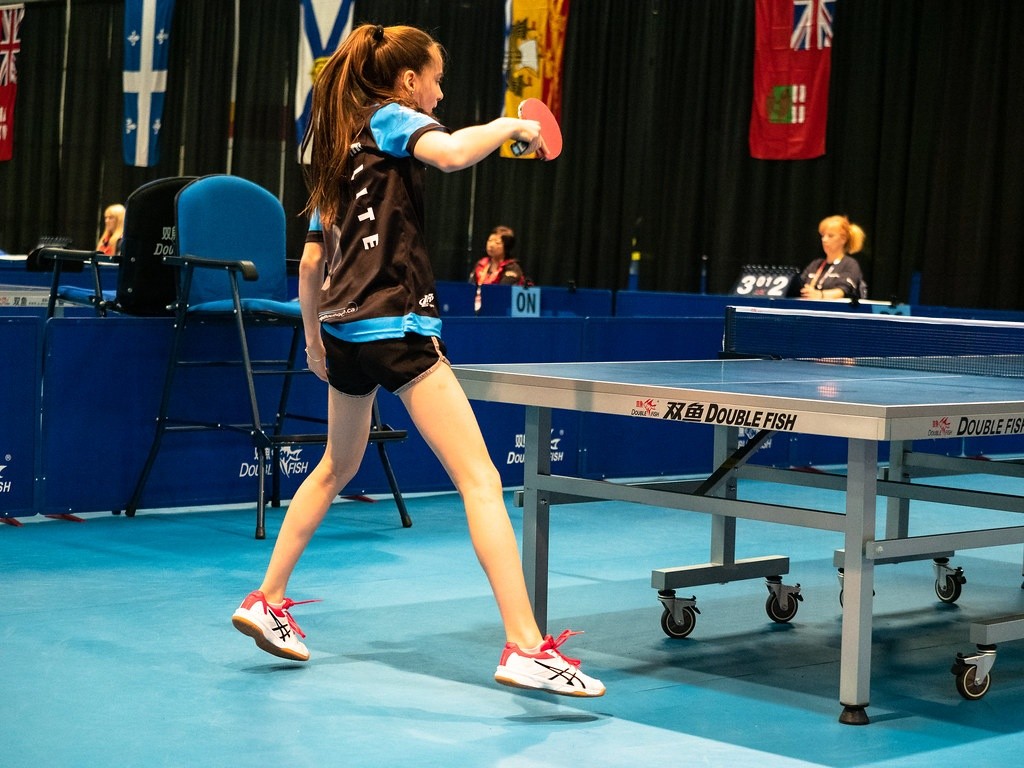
<box><xmin>819</xmin><ymin>289</ymin><xmax>825</xmax><ymax>299</ymax></box>
<box><xmin>304</xmin><ymin>346</ymin><xmax>327</xmax><ymax>361</ymax></box>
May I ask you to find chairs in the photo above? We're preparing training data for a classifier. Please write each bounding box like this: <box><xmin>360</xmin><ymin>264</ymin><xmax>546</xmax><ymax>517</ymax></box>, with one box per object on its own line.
<box><xmin>21</xmin><ymin>176</ymin><xmax>200</xmax><ymax>317</ymax></box>
<box><xmin>129</xmin><ymin>172</ymin><xmax>412</xmax><ymax>540</ymax></box>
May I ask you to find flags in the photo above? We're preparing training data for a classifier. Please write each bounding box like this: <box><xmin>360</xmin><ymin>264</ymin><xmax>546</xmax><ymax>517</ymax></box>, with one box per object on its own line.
<box><xmin>499</xmin><ymin>0</ymin><xmax>570</xmax><ymax>159</ymax></box>
<box><xmin>748</xmin><ymin>0</ymin><xmax>833</xmax><ymax>159</ymax></box>
<box><xmin>296</xmin><ymin>0</ymin><xmax>354</xmax><ymax>165</ymax></box>
<box><xmin>122</xmin><ymin>0</ymin><xmax>173</xmax><ymax>167</ymax></box>
<box><xmin>0</xmin><ymin>2</ymin><xmax>26</xmax><ymax>160</ymax></box>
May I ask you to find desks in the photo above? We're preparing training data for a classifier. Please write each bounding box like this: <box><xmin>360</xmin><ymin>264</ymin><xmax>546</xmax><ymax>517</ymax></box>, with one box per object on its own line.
<box><xmin>451</xmin><ymin>306</ymin><xmax>1024</xmax><ymax>727</ymax></box>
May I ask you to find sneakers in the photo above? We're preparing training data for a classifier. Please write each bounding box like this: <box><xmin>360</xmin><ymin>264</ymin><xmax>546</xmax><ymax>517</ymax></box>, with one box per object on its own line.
<box><xmin>232</xmin><ymin>590</ymin><xmax>311</xmax><ymax>662</ymax></box>
<box><xmin>494</xmin><ymin>628</ymin><xmax>607</xmax><ymax>698</ymax></box>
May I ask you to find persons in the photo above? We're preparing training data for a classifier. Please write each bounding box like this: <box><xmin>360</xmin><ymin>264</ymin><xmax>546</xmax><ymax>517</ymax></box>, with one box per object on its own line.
<box><xmin>95</xmin><ymin>202</ymin><xmax>126</xmax><ymax>255</ymax></box>
<box><xmin>231</xmin><ymin>22</ymin><xmax>606</xmax><ymax>698</ymax></box>
<box><xmin>467</xmin><ymin>225</ymin><xmax>527</xmax><ymax>287</ymax></box>
<box><xmin>800</xmin><ymin>215</ymin><xmax>863</xmax><ymax>301</ymax></box>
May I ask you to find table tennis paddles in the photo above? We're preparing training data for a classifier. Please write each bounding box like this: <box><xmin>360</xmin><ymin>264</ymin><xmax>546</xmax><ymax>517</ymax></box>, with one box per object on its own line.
<box><xmin>510</xmin><ymin>96</ymin><xmax>563</xmax><ymax>162</ymax></box>
